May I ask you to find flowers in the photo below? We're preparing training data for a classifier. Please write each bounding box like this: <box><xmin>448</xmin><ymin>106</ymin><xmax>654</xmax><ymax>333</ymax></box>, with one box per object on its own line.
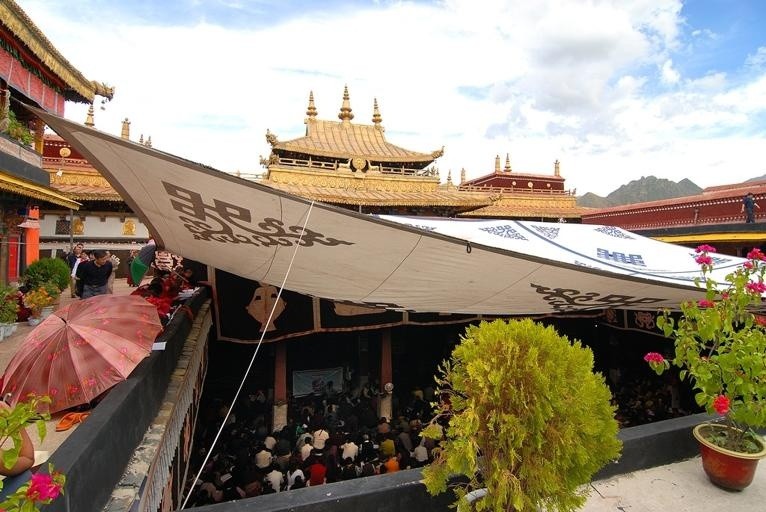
<box><xmin>645</xmin><ymin>243</ymin><xmax>766</xmax><ymax>446</ymax></box>
<box><xmin>26</xmin><ymin>286</ymin><xmax>52</xmax><ymax>316</ymax></box>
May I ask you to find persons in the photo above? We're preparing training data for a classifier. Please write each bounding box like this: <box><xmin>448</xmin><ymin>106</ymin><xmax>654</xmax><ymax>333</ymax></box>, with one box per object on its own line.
<box><xmin>131</xmin><ymin>262</ymin><xmax>211</xmax><ymax>328</ymax></box>
<box><xmin>741</xmin><ymin>192</ymin><xmax>761</xmax><ymax>223</ymax></box>
<box><xmin>601</xmin><ymin>370</ymin><xmax>699</xmax><ymax>429</ymax></box>
<box><xmin>0</xmin><ymin>400</ymin><xmax>35</xmax><ymax>478</ymax></box>
<box><xmin>125</xmin><ymin>250</ymin><xmax>138</xmax><ymax>287</ymax></box>
<box><xmin>61</xmin><ymin>244</ymin><xmax>120</xmax><ymax>301</ymax></box>
<box><xmin>182</xmin><ymin>372</ymin><xmax>452</xmax><ymax>508</ymax></box>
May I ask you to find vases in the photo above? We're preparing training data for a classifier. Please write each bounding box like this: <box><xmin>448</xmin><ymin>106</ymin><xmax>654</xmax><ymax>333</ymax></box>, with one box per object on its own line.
<box><xmin>28</xmin><ymin>306</ymin><xmax>54</xmax><ymax>326</ymax></box>
<box><xmin>693</xmin><ymin>422</ymin><xmax>766</xmax><ymax>492</ymax></box>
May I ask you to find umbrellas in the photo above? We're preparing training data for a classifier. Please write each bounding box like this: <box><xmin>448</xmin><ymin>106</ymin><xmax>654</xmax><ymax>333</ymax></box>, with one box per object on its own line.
<box><xmin>0</xmin><ymin>291</ymin><xmax>166</xmax><ymax>420</ymax></box>
<box><xmin>130</xmin><ymin>243</ymin><xmax>158</xmax><ymax>288</ymax></box>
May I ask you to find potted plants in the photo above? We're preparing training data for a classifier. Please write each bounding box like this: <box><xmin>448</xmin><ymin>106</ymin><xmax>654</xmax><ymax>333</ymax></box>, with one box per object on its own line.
<box><xmin>418</xmin><ymin>318</ymin><xmax>623</xmax><ymax>512</ymax></box>
<box><xmin>0</xmin><ymin>300</ymin><xmax>18</xmax><ymax>342</ymax></box>
